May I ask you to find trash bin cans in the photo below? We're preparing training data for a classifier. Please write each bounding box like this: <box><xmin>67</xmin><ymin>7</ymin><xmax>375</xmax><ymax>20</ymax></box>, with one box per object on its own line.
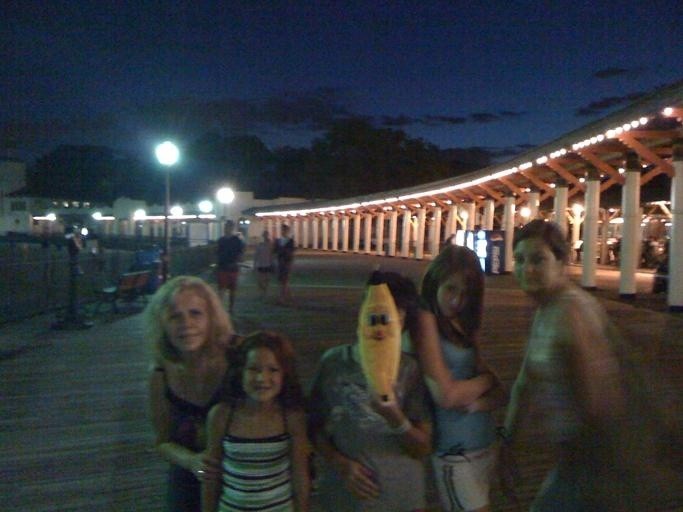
<box><xmin>135</xmin><ymin>250</ymin><xmax>162</xmax><ymax>293</ymax></box>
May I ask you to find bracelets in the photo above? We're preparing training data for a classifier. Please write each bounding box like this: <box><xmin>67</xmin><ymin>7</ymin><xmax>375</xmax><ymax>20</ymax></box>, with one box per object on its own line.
<box><xmin>384</xmin><ymin>420</ymin><xmax>412</xmax><ymax>437</ymax></box>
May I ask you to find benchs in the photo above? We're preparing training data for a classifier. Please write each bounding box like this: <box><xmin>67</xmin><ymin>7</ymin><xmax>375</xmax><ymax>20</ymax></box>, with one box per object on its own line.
<box><xmin>95</xmin><ymin>269</ymin><xmax>152</xmax><ymax>313</ymax></box>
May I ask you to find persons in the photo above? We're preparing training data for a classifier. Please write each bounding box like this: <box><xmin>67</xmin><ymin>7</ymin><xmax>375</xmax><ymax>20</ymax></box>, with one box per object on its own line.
<box><xmin>303</xmin><ymin>270</ymin><xmax>437</xmax><ymax>510</ymax></box>
<box><xmin>496</xmin><ymin>216</ymin><xmax>624</xmax><ymax>511</ymax></box>
<box><xmin>250</xmin><ymin>229</ymin><xmax>274</xmax><ymax>303</ymax></box>
<box><xmin>196</xmin><ymin>329</ymin><xmax>311</xmax><ymax>511</ymax></box>
<box><xmin>64</xmin><ymin>225</ymin><xmax>84</xmax><ymax>285</ymax></box>
<box><xmin>271</xmin><ymin>221</ymin><xmax>295</xmax><ymax>307</ymax></box>
<box><xmin>211</xmin><ymin>217</ymin><xmax>243</xmax><ymax>316</ymax></box>
<box><xmin>143</xmin><ymin>273</ymin><xmax>251</xmax><ymax>512</ymax></box>
<box><xmin>412</xmin><ymin>242</ymin><xmax>511</xmax><ymax>512</ymax></box>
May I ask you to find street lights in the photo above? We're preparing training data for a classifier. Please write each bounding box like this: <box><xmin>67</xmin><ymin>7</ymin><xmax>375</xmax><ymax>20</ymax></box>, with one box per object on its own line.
<box><xmin>198</xmin><ymin>200</ymin><xmax>213</xmax><ymax>242</ymax></box>
<box><xmin>459</xmin><ymin>210</ymin><xmax>467</xmax><ymax>229</ymax></box>
<box><xmin>46</xmin><ymin>213</ymin><xmax>57</xmax><ymax>235</ymax></box>
<box><xmin>216</xmin><ymin>187</ymin><xmax>234</xmax><ymax>238</ymax></box>
<box><xmin>570</xmin><ymin>204</ymin><xmax>582</xmax><ymax>260</ymax></box>
<box><xmin>170</xmin><ymin>207</ymin><xmax>183</xmax><ymax>239</ymax></box>
<box><xmin>155</xmin><ymin>142</ymin><xmax>178</xmax><ymax>289</ymax></box>
<box><xmin>520</xmin><ymin>207</ymin><xmax>530</xmax><ymax>226</ymax></box>
<box><xmin>92</xmin><ymin>211</ymin><xmax>100</xmax><ymax>235</ymax></box>
<box><xmin>134</xmin><ymin>210</ymin><xmax>145</xmax><ymax>239</ymax></box>
